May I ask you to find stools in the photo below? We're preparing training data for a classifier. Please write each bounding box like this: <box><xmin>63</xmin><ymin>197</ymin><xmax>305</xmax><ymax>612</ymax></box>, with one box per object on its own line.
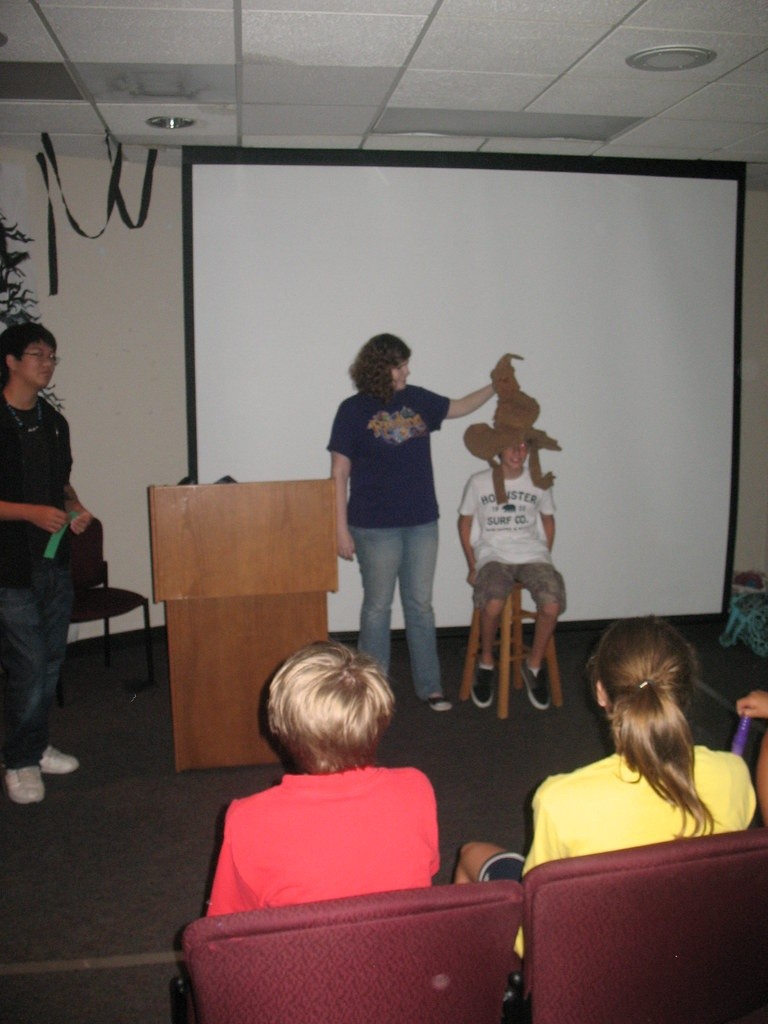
<box><xmin>458</xmin><ymin>580</ymin><xmax>565</xmax><ymax>721</ymax></box>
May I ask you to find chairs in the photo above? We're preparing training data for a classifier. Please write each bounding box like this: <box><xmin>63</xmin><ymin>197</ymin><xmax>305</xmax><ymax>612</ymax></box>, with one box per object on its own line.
<box><xmin>56</xmin><ymin>517</ymin><xmax>768</xmax><ymax>1024</ymax></box>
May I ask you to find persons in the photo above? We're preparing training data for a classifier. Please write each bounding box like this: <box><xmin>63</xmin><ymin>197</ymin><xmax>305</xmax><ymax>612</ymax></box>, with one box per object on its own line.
<box><xmin>326</xmin><ymin>334</ymin><xmax>496</xmax><ymax>711</ymax></box>
<box><xmin>458</xmin><ymin>439</ymin><xmax>567</xmax><ymax>710</ymax></box>
<box><xmin>452</xmin><ymin>612</ymin><xmax>757</xmax><ymax>958</ymax></box>
<box><xmin>206</xmin><ymin>640</ymin><xmax>441</xmax><ymax>915</ymax></box>
<box><xmin>736</xmin><ymin>689</ymin><xmax>768</xmax><ymax>829</ymax></box>
<box><xmin>0</xmin><ymin>323</ymin><xmax>93</xmax><ymax>804</ymax></box>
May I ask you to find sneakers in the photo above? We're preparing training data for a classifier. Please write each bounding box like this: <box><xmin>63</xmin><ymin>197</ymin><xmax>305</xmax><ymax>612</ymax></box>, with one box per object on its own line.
<box><xmin>39</xmin><ymin>745</ymin><xmax>80</xmax><ymax>775</ymax></box>
<box><xmin>5</xmin><ymin>765</ymin><xmax>45</xmax><ymax>804</ymax></box>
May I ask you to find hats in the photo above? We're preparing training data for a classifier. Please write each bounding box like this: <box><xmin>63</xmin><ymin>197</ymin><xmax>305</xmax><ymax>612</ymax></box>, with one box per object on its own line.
<box><xmin>463</xmin><ymin>353</ymin><xmax>563</xmax><ymax>505</ymax></box>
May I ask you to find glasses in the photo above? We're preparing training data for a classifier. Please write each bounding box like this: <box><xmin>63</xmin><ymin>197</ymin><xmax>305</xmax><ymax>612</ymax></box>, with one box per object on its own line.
<box><xmin>24</xmin><ymin>352</ymin><xmax>59</xmax><ymax>364</ymax></box>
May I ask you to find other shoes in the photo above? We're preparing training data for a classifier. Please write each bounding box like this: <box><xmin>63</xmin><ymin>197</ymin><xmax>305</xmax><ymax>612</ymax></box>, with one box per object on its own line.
<box><xmin>428</xmin><ymin>696</ymin><xmax>452</xmax><ymax>711</ymax></box>
<box><xmin>471</xmin><ymin>662</ymin><xmax>496</xmax><ymax>708</ymax></box>
<box><xmin>520</xmin><ymin>659</ymin><xmax>551</xmax><ymax>710</ymax></box>
